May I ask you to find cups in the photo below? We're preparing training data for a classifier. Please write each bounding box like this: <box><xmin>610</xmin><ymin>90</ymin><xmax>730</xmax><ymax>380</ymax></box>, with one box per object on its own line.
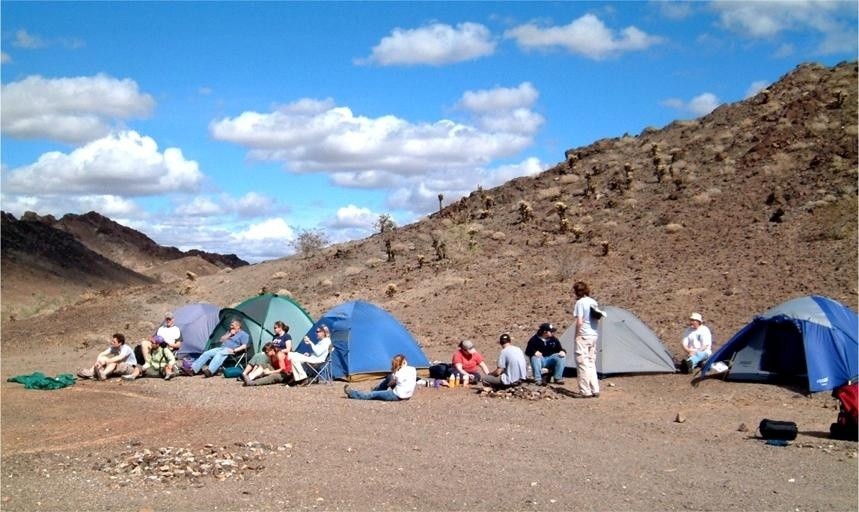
<box><xmin>425</xmin><ymin>380</ymin><xmax>430</xmax><ymax>387</ymax></box>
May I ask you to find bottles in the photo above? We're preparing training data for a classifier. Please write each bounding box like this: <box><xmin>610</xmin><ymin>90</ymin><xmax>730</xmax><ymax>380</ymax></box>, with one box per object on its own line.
<box><xmin>449</xmin><ymin>373</ymin><xmax>471</xmax><ymax>388</ymax></box>
<box><xmin>434</xmin><ymin>378</ymin><xmax>439</xmax><ymax>388</ymax></box>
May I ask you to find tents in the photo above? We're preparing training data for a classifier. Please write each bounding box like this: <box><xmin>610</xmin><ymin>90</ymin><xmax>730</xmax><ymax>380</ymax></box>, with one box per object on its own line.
<box><xmin>294</xmin><ymin>298</ymin><xmax>435</xmax><ymax>383</ymax></box>
<box><xmin>554</xmin><ymin>303</ymin><xmax>678</xmax><ymax>379</ymax></box>
<box><xmin>150</xmin><ymin>301</ymin><xmax>230</xmax><ymax>364</ymax></box>
<box><xmin>687</xmin><ymin>292</ymin><xmax>858</xmax><ymax>399</ymax></box>
<box><xmin>200</xmin><ymin>292</ymin><xmax>315</xmax><ymax>369</ymax></box>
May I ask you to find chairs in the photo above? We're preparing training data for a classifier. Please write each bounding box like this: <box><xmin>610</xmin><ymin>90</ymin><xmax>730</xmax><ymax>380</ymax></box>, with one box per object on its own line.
<box><xmin>302</xmin><ymin>344</ymin><xmax>336</xmax><ymax>387</ymax></box>
<box><xmin>171</xmin><ymin>349</ymin><xmax>179</xmax><ymax>374</ymax></box>
<box><xmin>227</xmin><ymin>346</ymin><xmax>248</xmax><ymax>371</ymax></box>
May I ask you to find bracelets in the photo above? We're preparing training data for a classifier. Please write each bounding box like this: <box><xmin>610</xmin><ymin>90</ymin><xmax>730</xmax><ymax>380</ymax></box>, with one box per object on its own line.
<box><xmin>233</xmin><ymin>349</ymin><xmax>235</xmax><ymax>353</ymax></box>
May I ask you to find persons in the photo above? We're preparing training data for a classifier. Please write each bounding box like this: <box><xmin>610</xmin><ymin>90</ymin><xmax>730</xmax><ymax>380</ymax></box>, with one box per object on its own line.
<box><xmin>679</xmin><ymin>311</ymin><xmax>713</xmax><ymax>374</ymax></box>
<box><xmin>286</xmin><ymin>323</ymin><xmax>332</xmax><ymax>387</ymax></box>
<box><xmin>180</xmin><ymin>318</ymin><xmax>250</xmax><ymax>378</ymax></box>
<box><xmin>481</xmin><ymin>333</ymin><xmax>527</xmax><ymax>392</ymax></box>
<box><xmin>524</xmin><ymin>322</ymin><xmax>567</xmax><ymax>386</ymax></box>
<box><xmin>120</xmin><ymin>334</ymin><xmax>177</xmax><ymax>381</ymax></box>
<box><xmin>156</xmin><ymin>311</ymin><xmax>184</xmax><ymax>357</ymax></box>
<box><xmin>343</xmin><ymin>354</ymin><xmax>417</xmax><ymax>401</ymax></box>
<box><xmin>572</xmin><ymin>281</ymin><xmax>601</xmax><ymax>399</ymax></box>
<box><xmin>77</xmin><ymin>333</ymin><xmax>138</xmax><ymax>381</ymax></box>
<box><xmin>239</xmin><ymin>342</ymin><xmax>292</xmax><ymax>386</ymax></box>
<box><xmin>236</xmin><ymin>320</ymin><xmax>292</xmax><ymax>383</ymax></box>
<box><xmin>452</xmin><ymin>339</ymin><xmax>490</xmax><ymax>385</ymax></box>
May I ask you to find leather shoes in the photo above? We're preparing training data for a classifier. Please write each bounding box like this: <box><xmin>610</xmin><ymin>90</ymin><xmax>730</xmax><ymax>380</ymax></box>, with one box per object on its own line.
<box><xmin>181</xmin><ymin>366</ymin><xmax>194</xmax><ymax>376</ymax></box>
<box><xmin>201</xmin><ymin>368</ymin><xmax>211</xmax><ymax>377</ymax></box>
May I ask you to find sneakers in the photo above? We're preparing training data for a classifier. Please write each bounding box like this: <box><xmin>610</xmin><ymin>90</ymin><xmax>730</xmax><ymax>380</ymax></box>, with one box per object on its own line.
<box><xmin>236</xmin><ymin>374</ymin><xmax>254</xmax><ymax>386</ymax></box>
<box><xmin>94</xmin><ymin>365</ymin><xmax>105</xmax><ymax>381</ymax></box>
<box><xmin>164</xmin><ymin>372</ymin><xmax>176</xmax><ymax>380</ymax></box>
<box><xmin>344</xmin><ymin>384</ymin><xmax>352</xmax><ymax>398</ymax></box>
<box><xmin>571</xmin><ymin>392</ymin><xmax>600</xmax><ymax>398</ymax></box>
<box><xmin>297</xmin><ymin>379</ymin><xmax>310</xmax><ymax>387</ymax></box>
<box><xmin>76</xmin><ymin>367</ymin><xmax>94</xmax><ymax>379</ymax></box>
<box><xmin>121</xmin><ymin>374</ymin><xmax>136</xmax><ymax>381</ymax></box>
<box><xmin>536</xmin><ymin>379</ymin><xmax>547</xmax><ymax>386</ymax></box>
<box><xmin>676</xmin><ymin>359</ymin><xmax>691</xmax><ymax>373</ymax></box>
<box><xmin>554</xmin><ymin>379</ymin><xmax>565</xmax><ymax>384</ymax></box>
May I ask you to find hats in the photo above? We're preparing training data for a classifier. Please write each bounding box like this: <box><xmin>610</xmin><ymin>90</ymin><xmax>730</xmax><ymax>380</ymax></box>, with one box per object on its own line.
<box><xmin>689</xmin><ymin>312</ymin><xmax>703</xmax><ymax>323</ymax></box>
<box><xmin>500</xmin><ymin>334</ymin><xmax>510</xmax><ymax>339</ymax></box>
<box><xmin>462</xmin><ymin>340</ymin><xmax>476</xmax><ymax>354</ymax></box>
<box><xmin>540</xmin><ymin>323</ymin><xmax>557</xmax><ymax>332</ymax></box>
<box><xmin>164</xmin><ymin>312</ymin><xmax>173</xmax><ymax>319</ymax></box>
<box><xmin>590</xmin><ymin>304</ymin><xmax>608</xmax><ymax>320</ymax></box>
